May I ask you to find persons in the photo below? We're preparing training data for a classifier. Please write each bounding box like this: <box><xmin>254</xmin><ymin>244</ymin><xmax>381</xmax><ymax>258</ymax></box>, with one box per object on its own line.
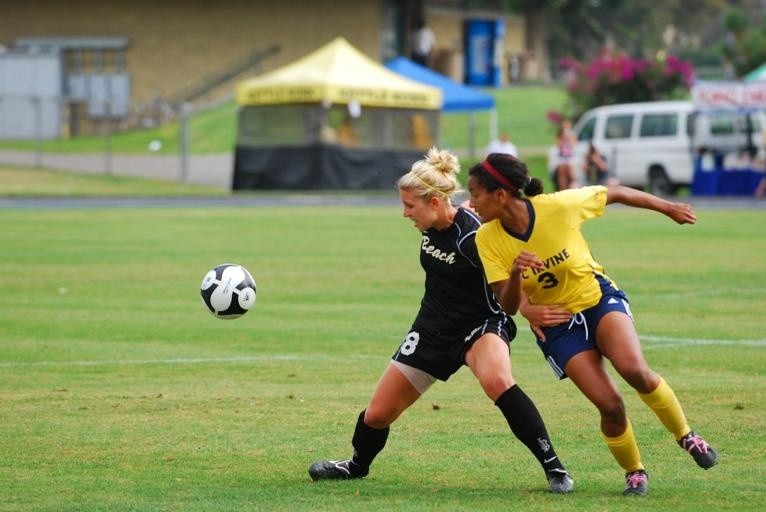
<box><xmin>556</xmin><ymin>119</ymin><xmax>577</xmax><ymax>190</ymax></box>
<box><xmin>307</xmin><ymin>147</ymin><xmax>575</xmax><ymax>493</ymax></box>
<box><xmin>485</xmin><ymin>132</ymin><xmax>517</xmax><ymax>158</ymax></box>
<box><xmin>403</xmin><ymin>20</ymin><xmax>436</xmax><ymax>68</ymax></box>
<box><xmin>468</xmin><ymin>153</ymin><xmax>717</xmax><ymax>495</ymax></box>
<box><xmin>581</xmin><ymin>145</ymin><xmax>618</xmax><ymax>187</ymax></box>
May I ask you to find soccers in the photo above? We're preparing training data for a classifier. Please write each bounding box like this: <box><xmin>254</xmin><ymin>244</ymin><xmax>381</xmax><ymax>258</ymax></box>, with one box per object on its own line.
<box><xmin>200</xmin><ymin>264</ymin><xmax>253</xmax><ymax>320</ymax></box>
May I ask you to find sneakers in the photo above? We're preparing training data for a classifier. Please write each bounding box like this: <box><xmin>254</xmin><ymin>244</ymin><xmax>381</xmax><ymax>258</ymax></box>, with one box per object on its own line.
<box><xmin>545</xmin><ymin>472</ymin><xmax>576</xmax><ymax>495</ymax></box>
<box><xmin>304</xmin><ymin>459</ymin><xmax>369</xmax><ymax>482</ymax></box>
<box><xmin>680</xmin><ymin>431</ymin><xmax>718</xmax><ymax>472</ymax></box>
<box><xmin>619</xmin><ymin>471</ymin><xmax>649</xmax><ymax>498</ymax></box>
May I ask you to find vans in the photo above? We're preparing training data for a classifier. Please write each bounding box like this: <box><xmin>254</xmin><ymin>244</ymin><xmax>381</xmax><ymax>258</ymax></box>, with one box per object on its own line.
<box><xmin>548</xmin><ymin>100</ymin><xmax>766</xmax><ymax>197</ymax></box>
